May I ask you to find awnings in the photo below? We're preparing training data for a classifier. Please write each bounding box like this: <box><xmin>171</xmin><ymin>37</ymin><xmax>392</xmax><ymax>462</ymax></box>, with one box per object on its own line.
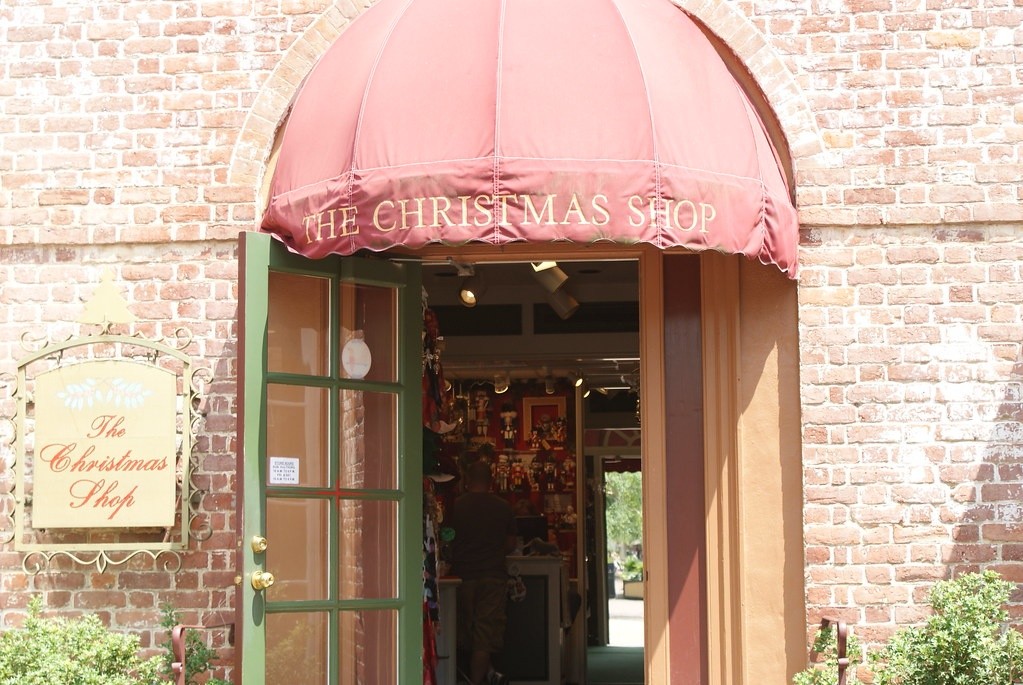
<box><xmin>261</xmin><ymin>0</ymin><xmax>803</xmax><ymax>282</ymax></box>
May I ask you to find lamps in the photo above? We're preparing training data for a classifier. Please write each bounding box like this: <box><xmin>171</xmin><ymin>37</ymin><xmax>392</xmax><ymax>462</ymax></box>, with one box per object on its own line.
<box><xmin>444</xmin><ymin>379</ymin><xmax>451</xmax><ymax>391</ymax></box>
<box><xmin>545</xmin><ymin>375</ymin><xmax>557</xmax><ymax>396</ymax></box>
<box><xmin>578</xmin><ymin>374</ymin><xmax>589</xmax><ymax>399</ymax></box>
<box><xmin>538</xmin><ymin>285</ymin><xmax>579</xmax><ymax>321</ymax></box>
<box><xmin>445</xmin><ymin>257</ymin><xmax>489</xmax><ymax>307</ymax></box>
<box><xmin>566</xmin><ymin>367</ymin><xmax>584</xmax><ymax>386</ymax></box>
<box><xmin>492</xmin><ymin>374</ymin><xmax>508</xmax><ymax>393</ymax></box>
<box><xmin>527</xmin><ymin>261</ymin><xmax>570</xmax><ymax>294</ymax></box>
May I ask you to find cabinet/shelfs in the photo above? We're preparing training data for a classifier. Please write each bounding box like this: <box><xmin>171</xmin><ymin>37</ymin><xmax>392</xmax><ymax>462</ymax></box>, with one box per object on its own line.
<box><xmin>433</xmin><ymin>576</ymin><xmax>461</xmax><ymax>685</ymax></box>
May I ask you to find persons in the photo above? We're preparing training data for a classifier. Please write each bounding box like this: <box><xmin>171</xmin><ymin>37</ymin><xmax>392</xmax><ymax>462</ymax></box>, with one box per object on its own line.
<box><xmin>446</xmin><ymin>461</ymin><xmax>519</xmax><ymax>685</ymax></box>
